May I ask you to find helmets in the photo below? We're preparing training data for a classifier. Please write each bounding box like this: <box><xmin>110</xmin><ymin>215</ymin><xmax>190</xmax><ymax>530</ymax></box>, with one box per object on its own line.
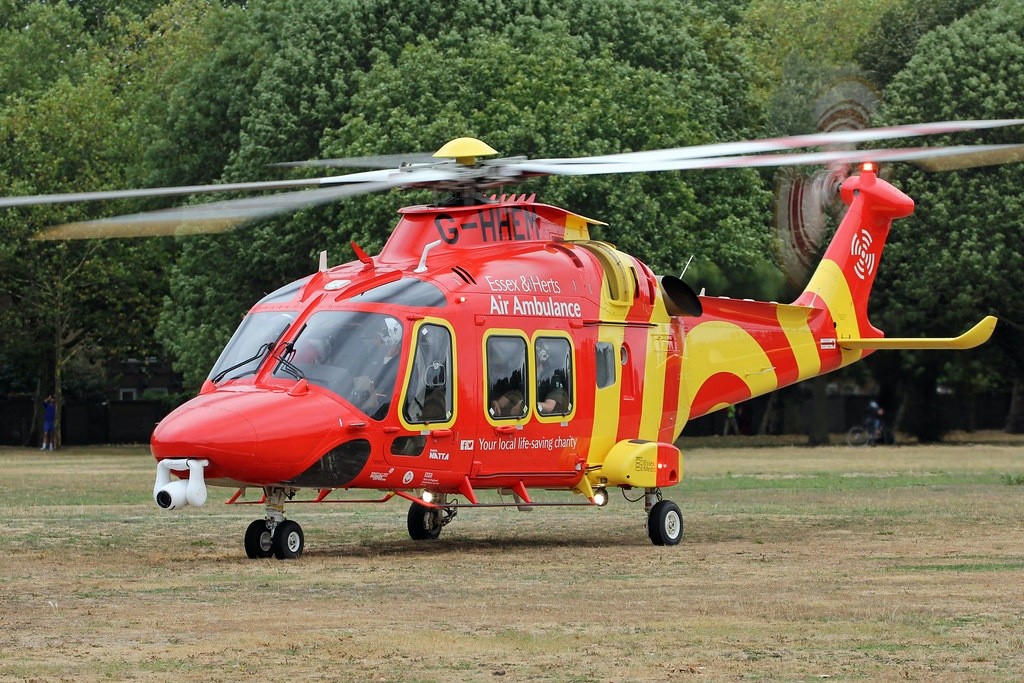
<box><xmin>376</xmin><ymin>318</ymin><xmax>402</xmax><ymax>345</ymax></box>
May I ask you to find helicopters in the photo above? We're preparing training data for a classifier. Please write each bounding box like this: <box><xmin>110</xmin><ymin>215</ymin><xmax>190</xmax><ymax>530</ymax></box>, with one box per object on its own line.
<box><xmin>1</xmin><ymin>114</ymin><xmax>1024</xmax><ymax>561</ymax></box>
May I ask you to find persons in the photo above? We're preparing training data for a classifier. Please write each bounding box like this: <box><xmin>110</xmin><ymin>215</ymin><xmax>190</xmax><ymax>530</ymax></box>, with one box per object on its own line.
<box><xmin>39</xmin><ymin>395</ymin><xmax>57</xmax><ymax>452</ymax></box>
<box><xmin>349</xmin><ymin>316</ymin><xmax>440</xmax><ymax>419</ymax></box>
<box><xmin>491</xmin><ymin>344</ymin><xmax>568</xmax><ymax>422</ymax></box>
